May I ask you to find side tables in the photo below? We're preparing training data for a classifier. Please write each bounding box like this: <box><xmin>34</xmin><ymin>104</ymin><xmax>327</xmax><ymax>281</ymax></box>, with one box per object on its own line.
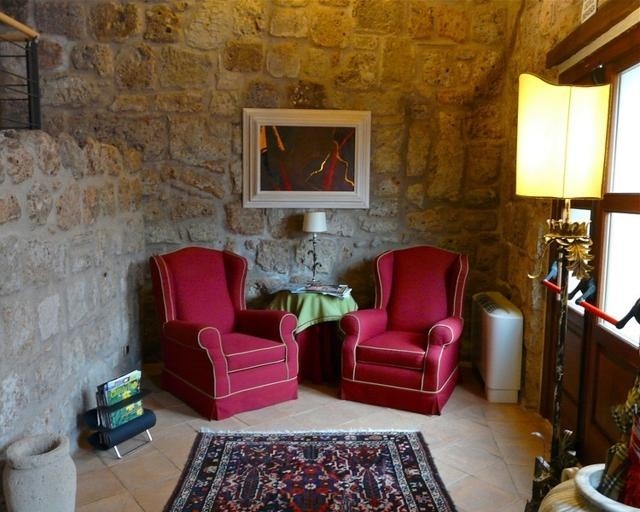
<box><xmin>266</xmin><ymin>291</ymin><xmax>359</xmax><ymax>386</ymax></box>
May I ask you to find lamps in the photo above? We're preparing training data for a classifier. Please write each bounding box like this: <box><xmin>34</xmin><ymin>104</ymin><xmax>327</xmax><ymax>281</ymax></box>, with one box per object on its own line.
<box><xmin>303</xmin><ymin>212</ymin><xmax>328</xmax><ymax>283</ymax></box>
<box><xmin>516</xmin><ymin>71</ymin><xmax>613</xmax><ymax>512</ymax></box>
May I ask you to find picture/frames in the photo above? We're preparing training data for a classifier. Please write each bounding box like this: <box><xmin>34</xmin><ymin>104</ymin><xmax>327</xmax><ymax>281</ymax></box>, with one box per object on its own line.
<box><xmin>241</xmin><ymin>107</ymin><xmax>369</xmax><ymax>210</ymax></box>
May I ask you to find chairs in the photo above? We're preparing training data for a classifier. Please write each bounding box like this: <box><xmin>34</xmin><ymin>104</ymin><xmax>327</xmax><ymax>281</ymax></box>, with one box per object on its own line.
<box><xmin>339</xmin><ymin>244</ymin><xmax>471</xmax><ymax>415</ymax></box>
<box><xmin>149</xmin><ymin>245</ymin><xmax>299</xmax><ymax>422</ymax></box>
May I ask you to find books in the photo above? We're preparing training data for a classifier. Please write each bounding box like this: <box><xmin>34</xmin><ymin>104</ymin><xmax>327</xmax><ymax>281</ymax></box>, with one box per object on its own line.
<box><xmin>289</xmin><ymin>284</ymin><xmax>352</xmax><ymax>300</ymax></box>
<box><xmin>97</xmin><ymin>369</ymin><xmax>142</xmax><ymax>429</ymax></box>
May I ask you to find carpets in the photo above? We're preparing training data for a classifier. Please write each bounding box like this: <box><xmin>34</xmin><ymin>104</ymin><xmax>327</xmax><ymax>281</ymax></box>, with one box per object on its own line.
<box><xmin>163</xmin><ymin>426</ymin><xmax>458</xmax><ymax>512</ymax></box>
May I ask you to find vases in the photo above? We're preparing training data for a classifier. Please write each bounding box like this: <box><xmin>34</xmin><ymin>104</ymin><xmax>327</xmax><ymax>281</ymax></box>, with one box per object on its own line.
<box><xmin>538</xmin><ymin>462</ymin><xmax>640</xmax><ymax>512</ymax></box>
<box><xmin>2</xmin><ymin>433</ymin><xmax>77</xmax><ymax>512</ymax></box>
